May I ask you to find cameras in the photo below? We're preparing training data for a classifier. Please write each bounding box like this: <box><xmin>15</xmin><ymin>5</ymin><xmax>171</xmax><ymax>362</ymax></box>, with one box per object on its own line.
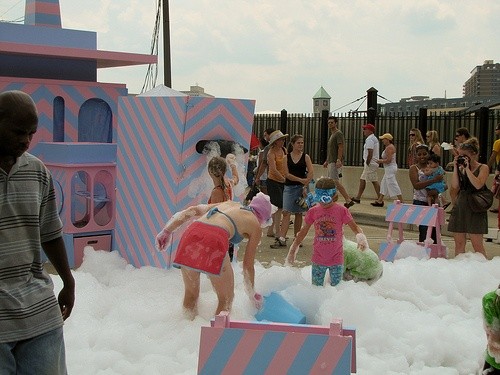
<box><xmin>457</xmin><ymin>154</ymin><xmax>466</xmax><ymax>165</ymax></box>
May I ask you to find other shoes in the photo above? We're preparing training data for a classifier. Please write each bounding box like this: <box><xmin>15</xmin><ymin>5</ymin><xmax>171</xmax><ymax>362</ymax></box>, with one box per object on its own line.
<box><xmin>350</xmin><ymin>198</ymin><xmax>360</xmax><ymax>203</ymax></box>
<box><xmin>293</xmin><ymin>237</ymin><xmax>303</xmax><ymax>247</ymax></box>
<box><xmin>270</xmin><ymin>237</ymin><xmax>287</xmax><ymax>249</ymax></box>
<box><xmin>343</xmin><ymin>201</ymin><xmax>354</xmax><ymax>208</ymax></box>
<box><xmin>280</xmin><ymin>220</ymin><xmax>294</xmax><ymax>226</ymax></box>
<box><xmin>370</xmin><ymin>201</ymin><xmax>384</xmax><ymax>207</ymax></box>
<box><xmin>489</xmin><ymin>208</ymin><xmax>499</xmax><ymax>214</ymax></box>
<box><xmin>267</xmin><ymin>233</ymin><xmax>276</xmax><ymax>238</ymax></box>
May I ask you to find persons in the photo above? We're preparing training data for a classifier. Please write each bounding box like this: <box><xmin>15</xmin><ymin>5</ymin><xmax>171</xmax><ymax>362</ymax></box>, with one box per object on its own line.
<box><xmin>322</xmin><ymin>116</ymin><xmax>355</xmax><ymax>209</ymax></box>
<box><xmin>265</xmin><ymin>130</ymin><xmax>290</xmax><ymax>240</ymax></box>
<box><xmin>269</xmin><ymin>134</ymin><xmax>314</xmax><ymax>249</ymax></box>
<box><xmin>350</xmin><ymin>124</ymin><xmax>385</xmax><ymax>205</ymax></box>
<box><xmin>247</xmin><ymin>126</ymin><xmax>276</xmax><ymax>195</ymax></box>
<box><xmin>488</xmin><ymin>122</ymin><xmax>500</xmax><ymax>244</ymax></box>
<box><xmin>449</xmin><ymin>127</ymin><xmax>472</xmax><ymax>157</ymax></box>
<box><xmin>155</xmin><ymin>194</ymin><xmax>272</xmax><ymax>320</ymax></box>
<box><xmin>206</xmin><ymin>155</ymin><xmax>240</xmax><ymax>267</ymax></box>
<box><xmin>480</xmin><ymin>281</ymin><xmax>500</xmax><ymax>375</ymax></box>
<box><xmin>409</xmin><ymin>128</ymin><xmax>450</xmax><ymax>243</ymax></box>
<box><xmin>0</xmin><ymin>90</ymin><xmax>75</xmax><ymax>375</ymax></box>
<box><xmin>370</xmin><ymin>133</ymin><xmax>403</xmax><ymax>207</ymax></box>
<box><xmin>452</xmin><ymin>138</ymin><xmax>490</xmax><ymax>258</ymax></box>
<box><xmin>285</xmin><ymin>176</ymin><xmax>367</xmax><ymax>287</ymax></box>
<box><xmin>341</xmin><ymin>232</ymin><xmax>383</xmax><ymax>284</ymax></box>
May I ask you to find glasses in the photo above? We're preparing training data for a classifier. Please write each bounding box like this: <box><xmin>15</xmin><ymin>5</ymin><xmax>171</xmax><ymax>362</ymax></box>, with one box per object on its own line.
<box><xmin>363</xmin><ymin>128</ymin><xmax>368</xmax><ymax>130</ymax></box>
<box><xmin>456</xmin><ymin>135</ymin><xmax>463</xmax><ymax>138</ymax></box>
<box><xmin>409</xmin><ymin>134</ymin><xmax>417</xmax><ymax>136</ymax></box>
<box><xmin>460</xmin><ymin>143</ymin><xmax>475</xmax><ymax>150</ymax></box>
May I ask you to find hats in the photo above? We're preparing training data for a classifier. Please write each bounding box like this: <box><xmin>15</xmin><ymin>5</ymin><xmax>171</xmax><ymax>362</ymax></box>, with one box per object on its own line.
<box><xmin>379</xmin><ymin>133</ymin><xmax>393</xmax><ymax>140</ymax></box>
<box><xmin>361</xmin><ymin>124</ymin><xmax>375</xmax><ymax>132</ymax></box>
<box><xmin>265</xmin><ymin>130</ymin><xmax>288</xmax><ymax>148</ymax></box>
<box><xmin>249</xmin><ymin>195</ymin><xmax>272</xmax><ymax>222</ymax></box>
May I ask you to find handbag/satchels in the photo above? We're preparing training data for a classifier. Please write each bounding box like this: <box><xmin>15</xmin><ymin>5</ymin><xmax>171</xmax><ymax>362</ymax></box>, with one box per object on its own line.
<box><xmin>439</xmin><ymin>191</ymin><xmax>451</xmax><ymax>210</ymax></box>
<box><xmin>295</xmin><ymin>194</ymin><xmax>312</xmax><ymax>210</ymax></box>
<box><xmin>474</xmin><ymin>187</ymin><xmax>493</xmax><ymax>210</ymax></box>
